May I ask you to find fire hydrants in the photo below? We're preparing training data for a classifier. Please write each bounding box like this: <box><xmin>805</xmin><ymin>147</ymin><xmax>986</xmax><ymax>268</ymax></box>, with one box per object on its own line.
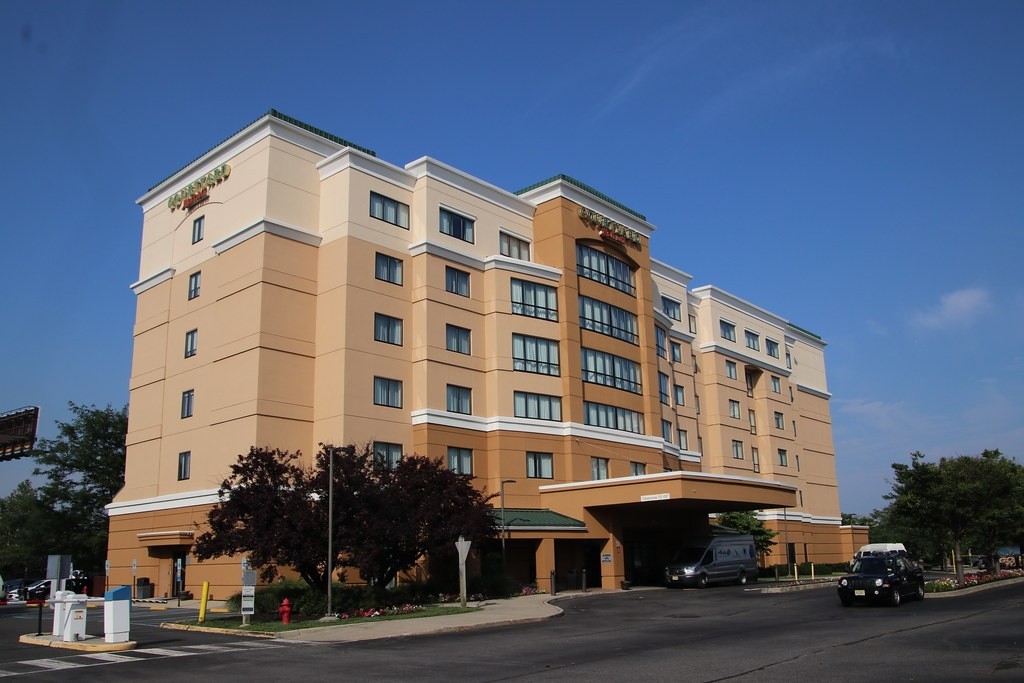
<box><xmin>277</xmin><ymin>598</ymin><xmax>292</xmax><ymax>625</ymax></box>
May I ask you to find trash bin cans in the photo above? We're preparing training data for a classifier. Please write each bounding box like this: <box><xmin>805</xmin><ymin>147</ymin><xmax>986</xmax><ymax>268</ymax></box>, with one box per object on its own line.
<box><xmin>137</xmin><ymin>577</ymin><xmax>150</xmax><ymax>599</ymax></box>
<box><xmin>621</xmin><ymin>581</ymin><xmax>630</xmax><ymax>590</ymax></box>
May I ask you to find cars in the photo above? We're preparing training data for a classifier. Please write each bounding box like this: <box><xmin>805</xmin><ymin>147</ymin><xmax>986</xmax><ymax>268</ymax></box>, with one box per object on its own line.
<box><xmin>26</xmin><ymin>581</ymin><xmax>88</xmax><ymax>601</ymax></box>
<box><xmin>838</xmin><ymin>554</ymin><xmax>924</xmax><ymax>609</ymax></box>
<box><xmin>6</xmin><ymin>579</ymin><xmax>52</xmax><ymax>602</ymax></box>
<box><xmin>0</xmin><ymin>575</ymin><xmax>6</xmax><ymax>599</ymax></box>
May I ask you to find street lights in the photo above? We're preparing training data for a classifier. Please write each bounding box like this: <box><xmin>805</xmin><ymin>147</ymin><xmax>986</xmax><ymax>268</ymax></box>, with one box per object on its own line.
<box><xmin>499</xmin><ymin>479</ymin><xmax>518</xmax><ymax>572</ymax></box>
<box><xmin>326</xmin><ymin>446</ymin><xmax>354</xmax><ymax>616</ymax></box>
<box><xmin>969</xmin><ymin>548</ymin><xmax>975</xmax><ymax>568</ymax></box>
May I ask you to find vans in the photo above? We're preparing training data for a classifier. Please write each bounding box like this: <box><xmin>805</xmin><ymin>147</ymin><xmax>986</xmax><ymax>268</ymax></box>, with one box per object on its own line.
<box><xmin>663</xmin><ymin>533</ymin><xmax>758</xmax><ymax>589</ymax></box>
<box><xmin>849</xmin><ymin>543</ymin><xmax>907</xmax><ymax>571</ymax></box>
